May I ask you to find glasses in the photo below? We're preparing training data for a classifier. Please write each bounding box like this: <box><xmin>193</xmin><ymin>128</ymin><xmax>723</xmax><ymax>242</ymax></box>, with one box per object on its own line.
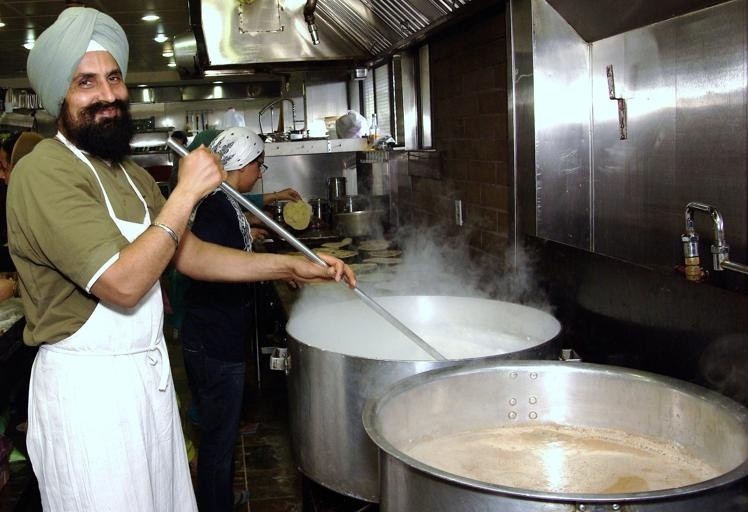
<box><xmin>254</xmin><ymin>159</ymin><xmax>268</xmax><ymax>173</ymax></box>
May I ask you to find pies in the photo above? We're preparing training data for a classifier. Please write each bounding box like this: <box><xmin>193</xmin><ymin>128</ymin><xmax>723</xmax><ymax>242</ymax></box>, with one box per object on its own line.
<box><xmin>283</xmin><ymin>200</ymin><xmax>312</xmax><ymax>231</ymax></box>
<box><xmin>308</xmin><ymin>238</ymin><xmax>407</xmax><ymax>291</ymax></box>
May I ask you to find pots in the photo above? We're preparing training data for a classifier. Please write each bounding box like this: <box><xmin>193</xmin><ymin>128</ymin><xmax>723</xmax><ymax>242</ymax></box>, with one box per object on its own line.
<box><xmin>360</xmin><ymin>359</ymin><xmax>746</xmax><ymax>508</ymax></box>
<box><xmin>269</xmin><ymin>295</ymin><xmax>560</xmax><ymax>507</ymax></box>
<box><xmin>333</xmin><ymin>207</ymin><xmax>387</xmax><ymax>238</ymax></box>
<box><xmin>172</xmin><ymin>1</ymin><xmax>205</xmax><ymax>80</ymax></box>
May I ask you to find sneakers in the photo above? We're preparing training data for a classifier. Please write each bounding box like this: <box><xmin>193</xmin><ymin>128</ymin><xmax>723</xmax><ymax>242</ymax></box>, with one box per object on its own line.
<box><xmin>236</xmin><ymin>422</ymin><xmax>273</xmax><ymax>447</ymax></box>
<box><xmin>191</xmin><ymin>425</ymin><xmax>243</xmax><ymax>470</ymax></box>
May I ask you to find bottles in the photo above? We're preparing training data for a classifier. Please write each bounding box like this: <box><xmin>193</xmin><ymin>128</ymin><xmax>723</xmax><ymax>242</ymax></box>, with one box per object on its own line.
<box><xmin>275</xmin><ymin>174</ymin><xmax>366</xmax><ymax>225</ymax></box>
<box><xmin>368</xmin><ymin>113</ymin><xmax>379</xmax><ymax>145</ymax></box>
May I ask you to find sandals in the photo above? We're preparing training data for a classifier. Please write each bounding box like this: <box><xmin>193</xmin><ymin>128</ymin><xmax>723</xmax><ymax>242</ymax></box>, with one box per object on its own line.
<box><xmin>232</xmin><ymin>486</ymin><xmax>251</xmax><ymax>509</ymax></box>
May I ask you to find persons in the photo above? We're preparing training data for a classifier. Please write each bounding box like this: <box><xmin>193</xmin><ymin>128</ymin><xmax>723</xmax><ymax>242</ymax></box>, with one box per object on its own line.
<box><xmin>180</xmin><ymin>127</ymin><xmax>270</xmax><ymax>512</ymax></box>
<box><xmin>7</xmin><ymin>7</ymin><xmax>357</xmax><ymax>512</ymax></box>
<box><xmin>0</xmin><ymin>130</ymin><xmax>46</xmax><ymax>302</ymax></box>
<box><xmin>166</xmin><ymin>129</ymin><xmax>301</xmax><ymax>424</ymax></box>
<box><xmin>169</xmin><ymin>131</ymin><xmax>188</xmax><ymax>195</ymax></box>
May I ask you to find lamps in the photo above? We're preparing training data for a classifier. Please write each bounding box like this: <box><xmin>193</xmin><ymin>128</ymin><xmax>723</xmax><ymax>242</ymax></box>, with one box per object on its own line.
<box><xmin>138</xmin><ymin>10</ymin><xmax>177</xmax><ymax>68</ymax></box>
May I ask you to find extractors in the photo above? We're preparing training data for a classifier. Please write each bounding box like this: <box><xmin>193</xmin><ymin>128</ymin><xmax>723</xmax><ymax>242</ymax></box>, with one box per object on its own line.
<box><xmin>307</xmin><ymin>0</ymin><xmax>471</xmax><ymax>64</ymax></box>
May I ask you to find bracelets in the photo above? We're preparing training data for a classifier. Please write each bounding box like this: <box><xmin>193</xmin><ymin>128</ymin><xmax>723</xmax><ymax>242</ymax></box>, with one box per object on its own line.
<box><xmin>150</xmin><ymin>223</ymin><xmax>179</xmax><ymax>250</ymax></box>
<box><xmin>274</xmin><ymin>191</ymin><xmax>278</xmax><ymax>204</ymax></box>
<box><xmin>7</xmin><ymin>274</ymin><xmax>17</xmax><ymax>295</ymax></box>
